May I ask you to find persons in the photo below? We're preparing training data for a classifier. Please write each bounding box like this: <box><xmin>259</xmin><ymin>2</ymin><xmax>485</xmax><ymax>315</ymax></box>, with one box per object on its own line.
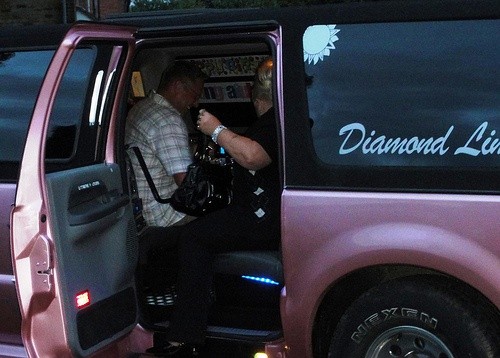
<box><xmin>143</xmin><ymin>58</ymin><xmax>281</xmax><ymax>358</ymax></box>
<box><xmin>121</xmin><ymin>53</ymin><xmax>210</xmax><ymax>230</ymax></box>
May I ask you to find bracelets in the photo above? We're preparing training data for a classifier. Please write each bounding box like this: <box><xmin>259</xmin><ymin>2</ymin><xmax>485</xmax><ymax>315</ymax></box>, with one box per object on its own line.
<box><xmin>211</xmin><ymin>125</ymin><xmax>228</xmax><ymax>145</ymax></box>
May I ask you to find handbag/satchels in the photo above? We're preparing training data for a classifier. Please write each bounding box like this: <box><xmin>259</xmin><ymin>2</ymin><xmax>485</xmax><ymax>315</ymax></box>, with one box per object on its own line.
<box><xmin>169</xmin><ymin>160</ymin><xmax>231</xmax><ymax>218</ymax></box>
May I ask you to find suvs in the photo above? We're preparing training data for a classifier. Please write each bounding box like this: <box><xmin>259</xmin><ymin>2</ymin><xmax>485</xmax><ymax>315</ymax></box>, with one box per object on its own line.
<box><xmin>1</xmin><ymin>10</ymin><xmax>499</xmax><ymax>358</ymax></box>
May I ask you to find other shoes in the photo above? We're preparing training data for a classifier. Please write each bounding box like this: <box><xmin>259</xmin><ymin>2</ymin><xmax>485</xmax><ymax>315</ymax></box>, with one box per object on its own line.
<box><xmin>146</xmin><ymin>343</ymin><xmax>200</xmax><ymax>357</ymax></box>
